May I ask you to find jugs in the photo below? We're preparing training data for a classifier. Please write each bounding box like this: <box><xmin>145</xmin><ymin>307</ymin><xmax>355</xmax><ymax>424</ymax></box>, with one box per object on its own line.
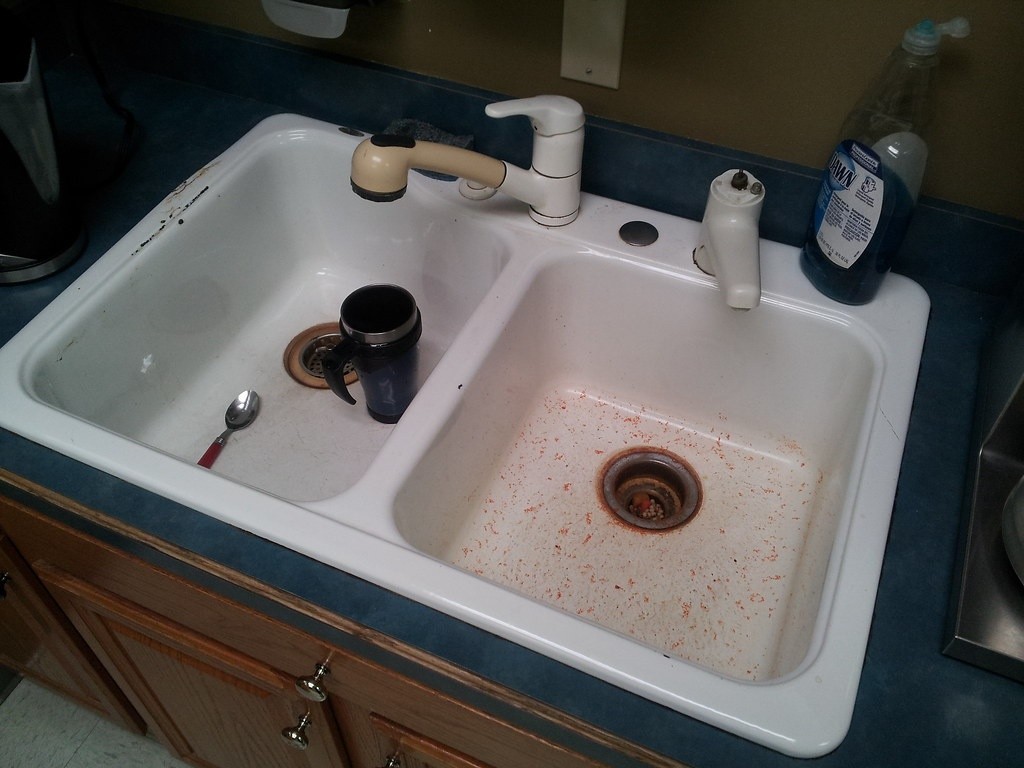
<box><xmin>0</xmin><ymin>27</ymin><xmax>87</xmax><ymax>288</ymax></box>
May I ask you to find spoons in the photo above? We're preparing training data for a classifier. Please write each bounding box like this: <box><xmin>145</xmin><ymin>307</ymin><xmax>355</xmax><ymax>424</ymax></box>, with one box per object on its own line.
<box><xmin>196</xmin><ymin>390</ymin><xmax>260</xmax><ymax>470</ymax></box>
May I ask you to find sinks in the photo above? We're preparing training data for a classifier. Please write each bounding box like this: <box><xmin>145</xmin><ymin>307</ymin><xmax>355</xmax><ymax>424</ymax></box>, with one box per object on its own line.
<box><xmin>18</xmin><ymin>128</ymin><xmax>520</xmax><ymax>514</ymax></box>
<box><xmin>378</xmin><ymin>241</ymin><xmax>889</xmax><ymax>689</ymax></box>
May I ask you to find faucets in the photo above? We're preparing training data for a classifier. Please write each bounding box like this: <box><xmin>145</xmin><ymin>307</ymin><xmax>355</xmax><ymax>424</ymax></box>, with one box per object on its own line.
<box><xmin>348</xmin><ymin>94</ymin><xmax>586</xmax><ymax>228</ymax></box>
<box><xmin>695</xmin><ymin>163</ymin><xmax>768</xmax><ymax>310</ymax></box>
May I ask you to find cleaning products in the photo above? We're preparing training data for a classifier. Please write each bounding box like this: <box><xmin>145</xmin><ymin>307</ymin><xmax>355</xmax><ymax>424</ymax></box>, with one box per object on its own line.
<box><xmin>797</xmin><ymin>15</ymin><xmax>976</xmax><ymax>309</ymax></box>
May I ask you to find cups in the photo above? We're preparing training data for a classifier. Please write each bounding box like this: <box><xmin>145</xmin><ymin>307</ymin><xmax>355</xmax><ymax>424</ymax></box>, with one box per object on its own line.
<box><xmin>320</xmin><ymin>283</ymin><xmax>423</xmax><ymax>425</ymax></box>
